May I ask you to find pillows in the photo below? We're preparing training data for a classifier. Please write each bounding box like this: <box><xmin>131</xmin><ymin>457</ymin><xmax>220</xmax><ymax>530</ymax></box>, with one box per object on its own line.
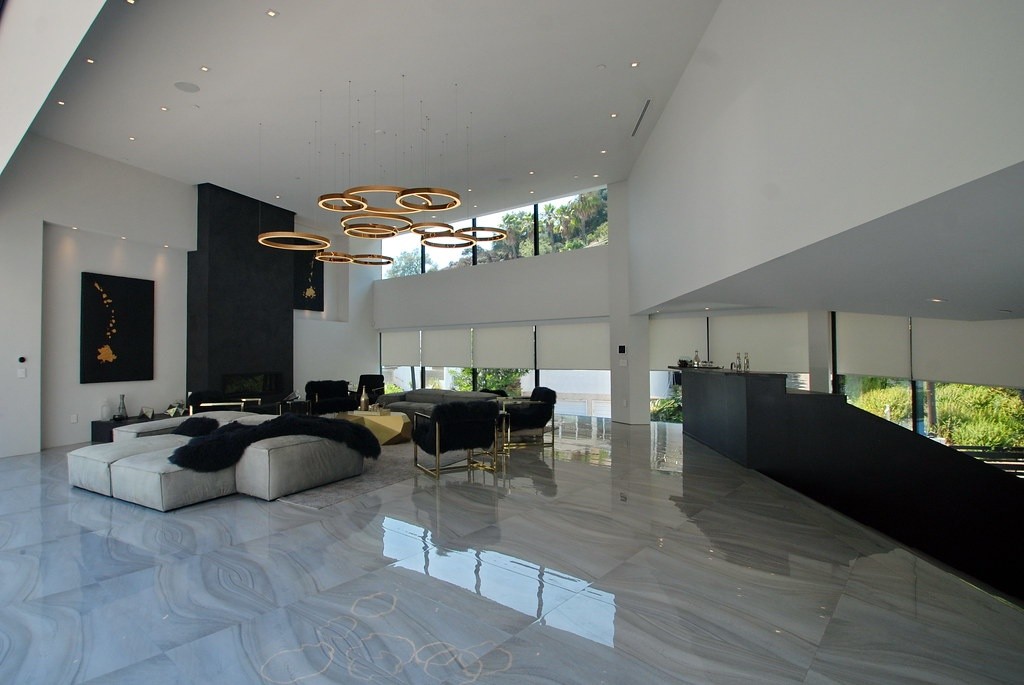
<box><xmin>170</xmin><ymin>417</ymin><xmax>219</xmax><ymax>437</ymax></box>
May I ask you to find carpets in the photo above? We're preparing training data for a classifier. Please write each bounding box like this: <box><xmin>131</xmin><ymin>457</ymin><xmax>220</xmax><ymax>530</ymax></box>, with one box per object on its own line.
<box><xmin>277</xmin><ymin>439</ymin><xmax>506</xmax><ymax>510</ymax></box>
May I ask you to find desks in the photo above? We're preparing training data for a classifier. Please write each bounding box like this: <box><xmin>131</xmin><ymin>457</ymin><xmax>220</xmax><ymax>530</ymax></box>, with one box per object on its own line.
<box><xmin>668</xmin><ymin>366</ymin><xmax>847</xmax><ymax>469</ymax></box>
<box><xmin>91</xmin><ymin>413</ymin><xmax>170</xmax><ymax>445</ymax></box>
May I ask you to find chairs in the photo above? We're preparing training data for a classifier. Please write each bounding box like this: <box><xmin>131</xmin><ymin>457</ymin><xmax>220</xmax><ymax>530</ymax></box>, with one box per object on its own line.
<box><xmin>188</xmin><ymin>372</ymin><xmax>385</xmax><ymax>415</ymax></box>
<box><xmin>412</xmin><ymin>387</ymin><xmax>556</xmax><ymax>479</ymax></box>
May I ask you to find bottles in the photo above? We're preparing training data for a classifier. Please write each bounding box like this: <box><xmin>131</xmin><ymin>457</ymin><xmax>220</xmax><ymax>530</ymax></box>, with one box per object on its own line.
<box><xmin>744</xmin><ymin>352</ymin><xmax>750</xmax><ymax>371</ymax></box>
<box><xmin>693</xmin><ymin>350</ymin><xmax>700</xmax><ymax>367</ymax></box>
<box><xmin>736</xmin><ymin>352</ymin><xmax>741</xmax><ymax>372</ymax></box>
<box><xmin>118</xmin><ymin>394</ymin><xmax>127</xmax><ymax>418</ymax></box>
<box><xmin>360</xmin><ymin>385</ymin><xmax>369</xmax><ymax>411</ymax></box>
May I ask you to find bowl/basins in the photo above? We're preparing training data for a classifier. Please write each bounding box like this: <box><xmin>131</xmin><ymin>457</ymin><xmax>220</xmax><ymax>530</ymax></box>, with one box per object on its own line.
<box><xmin>380</xmin><ymin>408</ymin><xmax>390</xmax><ymax>416</ymax></box>
<box><xmin>113</xmin><ymin>415</ymin><xmax>124</xmax><ymax>421</ymax></box>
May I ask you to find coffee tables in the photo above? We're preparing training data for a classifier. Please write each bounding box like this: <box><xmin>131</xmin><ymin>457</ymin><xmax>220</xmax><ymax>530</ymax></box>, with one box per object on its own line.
<box><xmin>335</xmin><ymin>412</ymin><xmax>412</xmax><ymax>445</ymax></box>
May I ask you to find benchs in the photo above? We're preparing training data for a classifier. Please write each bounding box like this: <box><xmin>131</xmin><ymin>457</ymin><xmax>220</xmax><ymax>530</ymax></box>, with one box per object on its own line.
<box><xmin>67</xmin><ymin>389</ymin><xmax>381</xmax><ymax>512</ymax></box>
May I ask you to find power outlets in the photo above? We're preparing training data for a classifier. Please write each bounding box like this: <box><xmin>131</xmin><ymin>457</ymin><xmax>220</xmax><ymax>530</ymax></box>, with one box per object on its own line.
<box><xmin>71</xmin><ymin>415</ymin><xmax>78</xmax><ymax>423</ymax></box>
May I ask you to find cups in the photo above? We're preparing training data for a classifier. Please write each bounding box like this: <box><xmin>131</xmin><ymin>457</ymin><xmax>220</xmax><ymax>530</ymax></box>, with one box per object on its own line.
<box><xmin>368</xmin><ymin>403</ymin><xmax>379</xmax><ymax>412</ymax></box>
<box><xmin>701</xmin><ymin>361</ymin><xmax>714</xmax><ymax>367</ymax></box>
<box><xmin>679</xmin><ymin>356</ymin><xmax>690</xmax><ymax>364</ymax></box>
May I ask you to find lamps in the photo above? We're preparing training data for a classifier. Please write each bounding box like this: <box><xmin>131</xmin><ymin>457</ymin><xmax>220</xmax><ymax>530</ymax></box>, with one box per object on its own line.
<box><xmin>257</xmin><ymin>74</ymin><xmax>509</xmax><ymax>267</ymax></box>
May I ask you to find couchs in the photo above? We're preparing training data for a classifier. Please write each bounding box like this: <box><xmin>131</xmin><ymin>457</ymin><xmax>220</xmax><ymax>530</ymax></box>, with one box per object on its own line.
<box><xmin>376</xmin><ymin>389</ymin><xmax>500</xmax><ymax>424</ymax></box>
